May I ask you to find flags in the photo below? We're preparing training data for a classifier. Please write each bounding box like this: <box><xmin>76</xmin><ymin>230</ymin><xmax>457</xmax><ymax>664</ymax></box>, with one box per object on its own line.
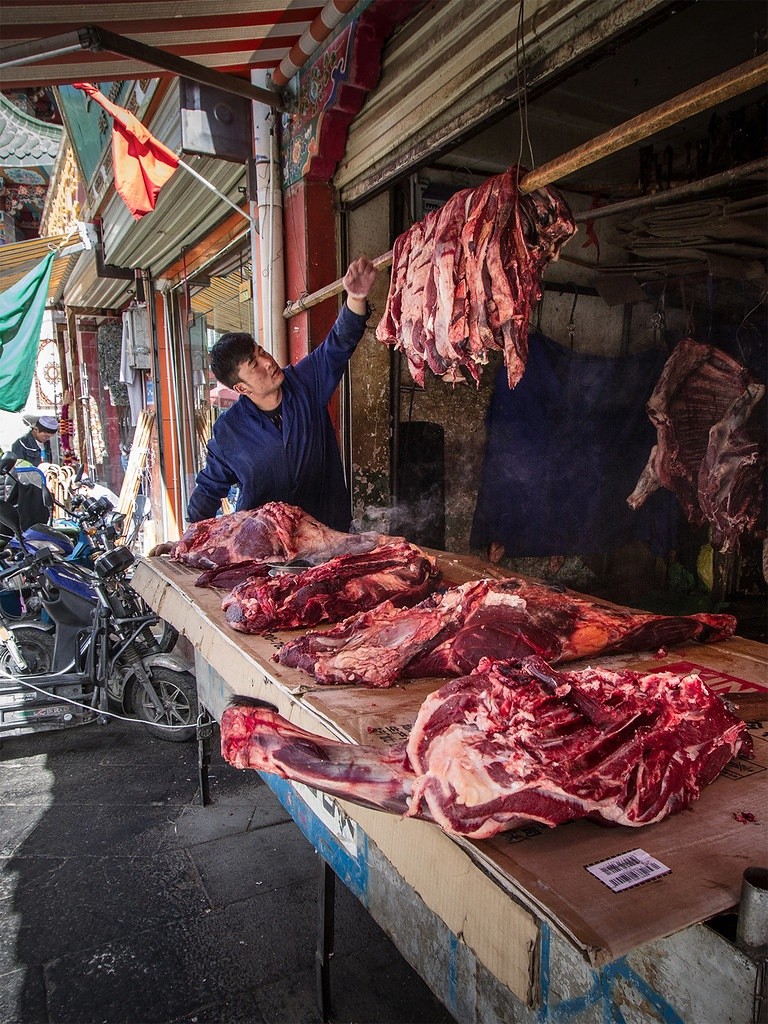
<box><xmin>73</xmin><ymin>80</ymin><xmax>180</xmax><ymax>221</ymax></box>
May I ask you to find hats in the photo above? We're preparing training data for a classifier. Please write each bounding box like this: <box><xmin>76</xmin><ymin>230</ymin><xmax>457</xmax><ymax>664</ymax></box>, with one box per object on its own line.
<box><xmin>38</xmin><ymin>417</ymin><xmax>59</xmax><ymax>430</ymax></box>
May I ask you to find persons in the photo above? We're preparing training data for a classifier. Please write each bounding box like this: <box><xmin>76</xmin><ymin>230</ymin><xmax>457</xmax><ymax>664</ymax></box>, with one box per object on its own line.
<box><xmin>148</xmin><ymin>256</ymin><xmax>378</xmax><ymax>557</ymax></box>
<box><xmin>12</xmin><ymin>416</ymin><xmax>58</xmax><ymax>467</ymax></box>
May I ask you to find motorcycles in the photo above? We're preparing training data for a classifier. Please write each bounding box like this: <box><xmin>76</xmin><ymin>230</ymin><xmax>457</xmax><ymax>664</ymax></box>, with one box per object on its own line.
<box><xmin>1</xmin><ymin>451</ymin><xmax>198</xmax><ymax>743</ymax></box>
